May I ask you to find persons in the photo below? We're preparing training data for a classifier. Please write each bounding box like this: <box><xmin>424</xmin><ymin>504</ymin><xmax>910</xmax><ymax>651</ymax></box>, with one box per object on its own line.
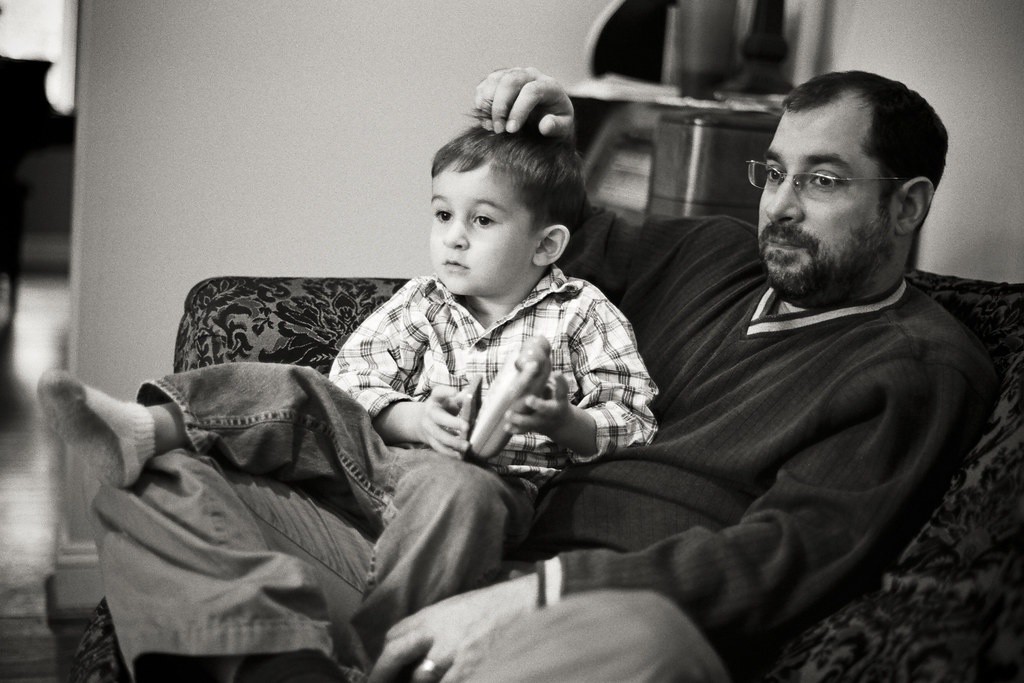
<box><xmin>38</xmin><ymin>100</ymin><xmax>658</xmax><ymax>661</ymax></box>
<box><xmin>92</xmin><ymin>64</ymin><xmax>997</xmax><ymax>683</ymax></box>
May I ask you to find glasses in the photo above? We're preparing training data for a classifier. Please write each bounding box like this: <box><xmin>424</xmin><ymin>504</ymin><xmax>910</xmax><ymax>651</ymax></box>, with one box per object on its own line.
<box><xmin>744</xmin><ymin>158</ymin><xmax>900</xmax><ymax>200</ymax></box>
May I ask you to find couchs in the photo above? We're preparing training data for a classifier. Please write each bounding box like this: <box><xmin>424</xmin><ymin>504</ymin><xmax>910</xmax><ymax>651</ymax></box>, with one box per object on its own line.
<box><xmin>75</xmin><ymin>271</ymin><xmax>1024</xmax><ymax>683</ymax></box>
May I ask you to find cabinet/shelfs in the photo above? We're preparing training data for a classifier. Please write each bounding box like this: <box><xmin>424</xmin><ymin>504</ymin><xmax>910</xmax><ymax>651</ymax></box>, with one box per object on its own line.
<box><xmin>568</xmin><ymin>93</ymin><xmax>780</xmax><ymax>231</ymax></box>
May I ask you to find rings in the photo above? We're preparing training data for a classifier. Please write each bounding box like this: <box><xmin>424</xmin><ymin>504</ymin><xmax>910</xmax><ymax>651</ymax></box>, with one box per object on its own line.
<box><xmin>420</xmin><ymin>658</ymin><xmax>445</xmax><ymax>680</ymax></box>
